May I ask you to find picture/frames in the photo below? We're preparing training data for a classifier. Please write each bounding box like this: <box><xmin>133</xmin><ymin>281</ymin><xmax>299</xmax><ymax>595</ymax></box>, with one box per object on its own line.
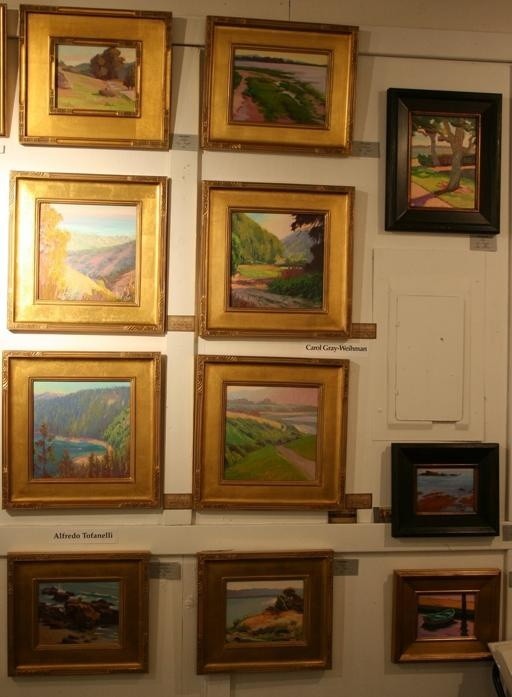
<box><xmin>383</xmin><ymin>88</ymin><xmax>501</xmax><ymax>235</ymax></box>
<box><xmin>18</xmin><ymin>5</ymin><xmax>173</xmax><ymax>152</ymax></box>
<box><xmin>391</xmin><ymin>442</ymin><xmax>500</xmax><ymax>537</ymax></box>
<box><xmin>200</xmin><ymin>15</ymin><xmax>360</xmax><ymax>152</ymax></box>
<box><xmin>195</xmin><ymin>549</ymin><xmax>334</xmax><ymax>675</ymax></box>
<box><xmin>4</xmin><ymin>350</ymin><xmax>163</xmax><ymax>512</ymax></box>
<box><xmin>391</xmin><ymin>569</ymin><xmax>505</xmax><ymax>663</ymax></box>
<box><xmin>7</xmin><ymin>170</ymin><xmax>167</xmax><ymax>335</ymax></box>
<box><xmin>7</xmin><ymin>551</ymin><xmax>150</xmax><ymax>675</ymax></box>
<box><xmin>200</xmin><ymin>180</ymin><xmax>355</xmax><ymax>339</ymax></box>
<box><xmin>191</xmin><ymin>356</ymin><xmax>349</xmax><ymax>514</ymax></box>
